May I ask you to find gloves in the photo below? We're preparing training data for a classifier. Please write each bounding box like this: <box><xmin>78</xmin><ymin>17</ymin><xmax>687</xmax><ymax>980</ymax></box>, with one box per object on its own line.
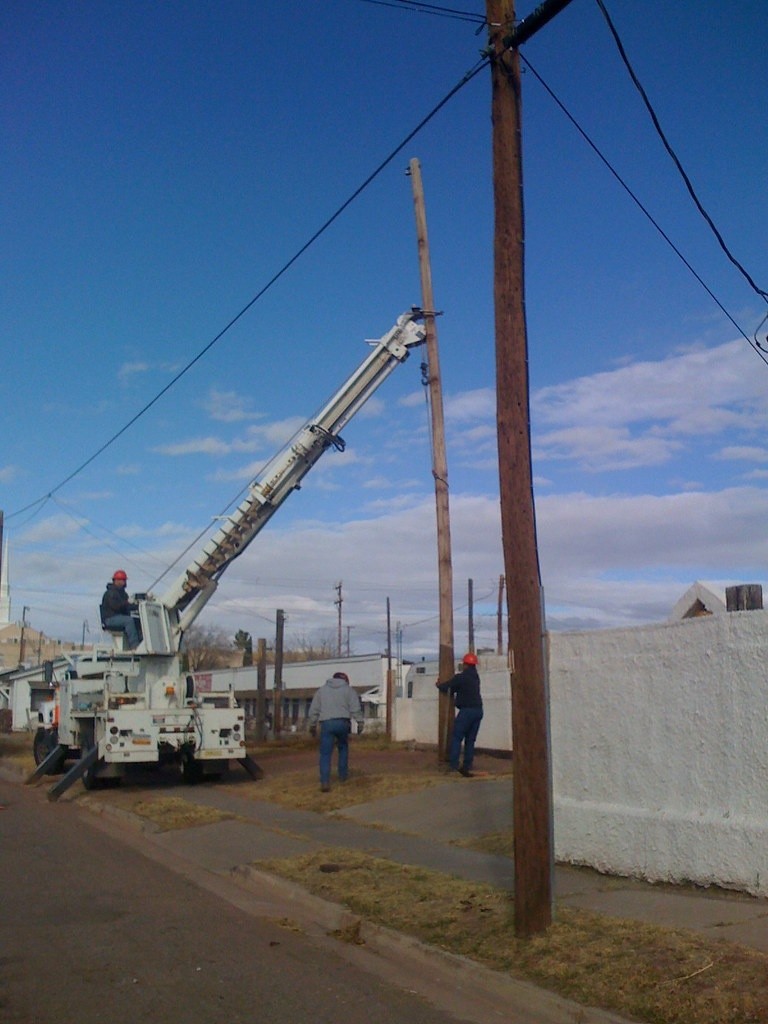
<box><xmin>310</xmin><ymin>726</ymin><xmax>316</xmax><ymax>735</ymax></box>
<box><xmin>357</xmin><ymin>721</ymin><xmax>364</xmax><ymax>734</ymax></box>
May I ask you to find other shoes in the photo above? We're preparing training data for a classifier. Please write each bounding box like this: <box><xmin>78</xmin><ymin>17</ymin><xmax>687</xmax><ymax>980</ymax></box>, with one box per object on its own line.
<box><xmin>320</xmin><ymin>784</ymin><xmax>330</xmax><ymax>791</ymax></box>
<box><xmin>445</xmin><ymin>765</ymin><xmax>459</xmax><ymax>771</ymax></box>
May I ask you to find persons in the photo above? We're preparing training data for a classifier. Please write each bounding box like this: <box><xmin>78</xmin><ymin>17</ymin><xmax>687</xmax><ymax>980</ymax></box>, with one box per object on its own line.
<box><xmin>99</xmin><ymin>569</ymin><xmax>139</xmax><ymax>652</ymax></box>
<box><xmin>310</xmin><ymin>672</ymin><xmax>364</xmax><ymax>792</ymax></box>
<box><xmin>436</xmin><ymin>653</ymin><xmax>484</xmax><ymax>777</ymax></box>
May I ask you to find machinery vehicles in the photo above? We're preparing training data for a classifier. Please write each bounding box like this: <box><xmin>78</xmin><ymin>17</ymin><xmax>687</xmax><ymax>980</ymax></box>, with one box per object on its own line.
<box><xmin>23</xmin><ymin>301</ymin><xmax>445</xmax><ymax>803</ymax></box>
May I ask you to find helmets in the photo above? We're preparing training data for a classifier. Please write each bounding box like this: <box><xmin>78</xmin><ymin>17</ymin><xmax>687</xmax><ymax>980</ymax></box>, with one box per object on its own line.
<box><xmin>333</xmin><ymin>672</ymin><xmax>349</xmax><ymax>684</ymax></box>
<box><xmin>113</xmin><ymin>571</ymin><xmax>128</xmax><ymax>580</ymax></box>
<box><xmin>463</xmin><ymin>654</ymin><xmax>479</xmax><ymax>665</ymax></box>
